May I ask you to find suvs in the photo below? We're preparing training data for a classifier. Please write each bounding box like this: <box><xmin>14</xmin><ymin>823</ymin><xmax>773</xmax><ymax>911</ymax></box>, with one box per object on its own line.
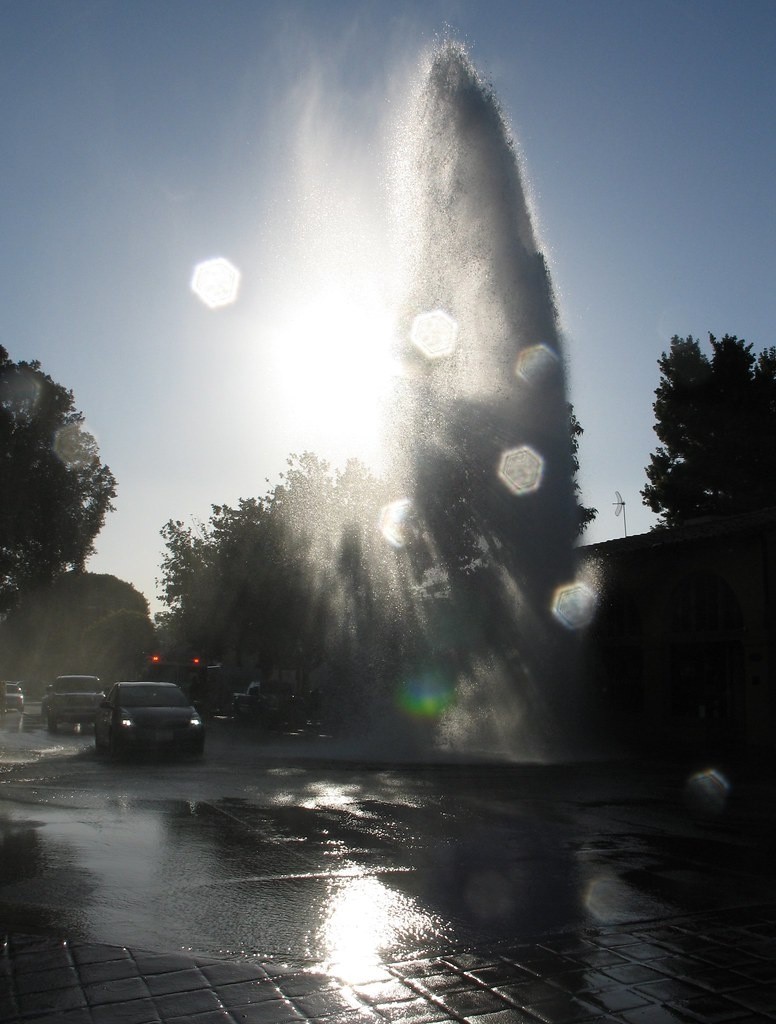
<box><xmin>41</xmin><ymin>675</ymin><xmax>107</xmax><ymax>733</ymax></box>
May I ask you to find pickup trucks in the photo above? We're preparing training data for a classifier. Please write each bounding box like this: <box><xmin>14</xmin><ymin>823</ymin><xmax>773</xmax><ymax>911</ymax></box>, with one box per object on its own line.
<box><xmin>232</xmin><ymin>680</ymin><xmax>292</xmax><ymax>729</ymax></box>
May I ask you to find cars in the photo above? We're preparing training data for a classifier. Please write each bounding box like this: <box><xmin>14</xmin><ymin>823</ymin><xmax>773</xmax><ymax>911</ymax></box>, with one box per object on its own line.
<box><xmin>4</xmin><ymin>681</ymin><xmax>23</xmax><ymax>713</ymax></box>
<box><xmin>94</xmin><ymin>681</ymin><xmax>204</xmax><ymax>763</ymax></box>
